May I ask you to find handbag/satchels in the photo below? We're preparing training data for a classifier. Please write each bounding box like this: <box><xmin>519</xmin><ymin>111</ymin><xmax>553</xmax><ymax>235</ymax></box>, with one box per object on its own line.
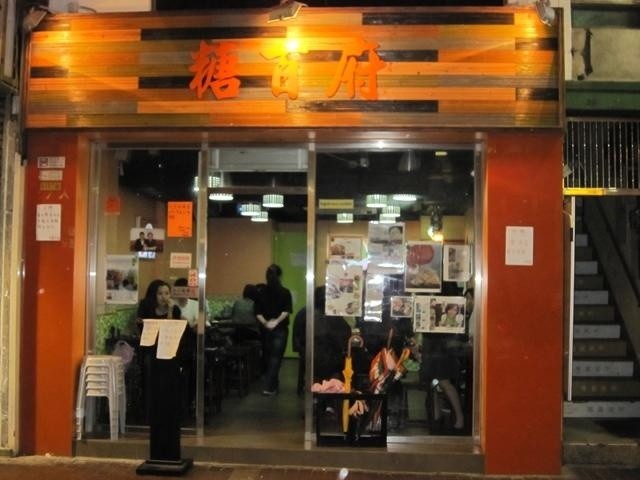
<box><xmin>112</xmin><ymin>341</ymin><xmax>135</xmax><ymax>372</ymax></box>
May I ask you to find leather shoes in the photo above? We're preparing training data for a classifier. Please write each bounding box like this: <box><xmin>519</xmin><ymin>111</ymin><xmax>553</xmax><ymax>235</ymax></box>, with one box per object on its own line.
<box><xmin>453</xmin><ymin>415</ymin><xmax>466</xmax><ymax>433</ymax></box>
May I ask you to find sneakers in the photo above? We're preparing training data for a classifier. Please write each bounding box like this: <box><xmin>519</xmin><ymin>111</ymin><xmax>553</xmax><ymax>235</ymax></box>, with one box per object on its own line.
<box><xmin>262</xmin><ymin>383</ymin><xmax>278</xmax><ymax>396</ymax></box>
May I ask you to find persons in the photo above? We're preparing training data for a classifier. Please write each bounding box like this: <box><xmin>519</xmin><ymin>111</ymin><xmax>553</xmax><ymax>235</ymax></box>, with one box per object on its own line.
<box><xmin>232</xmin><ymin>264</ymin><xmax>293</xmax><ymax>395</ymax></box>
<box><xmin>143</xmin><ymin>232</ymin><xmax>158</xmax><ymax>251</ymax></box>
<box><xmin>293</xmin><ymin>277</ymin><xmax>474</xmax><ymax>437</ymax></box>
<box><xmin>387</xmin><ymin>226</ymin><xmax>404</xmax><ymax>246</ymax></box>
<box><xmin>131</xmin><ymin>277</ymin><xmax>211</xmax><ymax>331</ymax></box>
<box><xmin>134</xmin><ymin>231</ymin><xmax>148</xmax><ymax>251</ymax></box>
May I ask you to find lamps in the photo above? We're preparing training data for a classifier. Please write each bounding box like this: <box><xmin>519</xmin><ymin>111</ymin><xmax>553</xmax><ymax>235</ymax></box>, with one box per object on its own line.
<box><xmin>192</xmin><ymin>149</ymin><xmax>285</xmax><ymax>223</ymax></box>
<box><xmin>336</xmin><ymin>193</ymin><xmax>418</xmax><ymax>225</ymax></box>
<box><xmin>397</xmin><ymin>149</ymin><xmax>422</xmax><ymax>173</ymax></box>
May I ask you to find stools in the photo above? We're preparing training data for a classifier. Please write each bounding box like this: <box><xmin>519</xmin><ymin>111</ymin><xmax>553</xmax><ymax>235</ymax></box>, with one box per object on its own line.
<box><xmin>73</xmin><ymin>352</ymin><xmax>127</xmax><ymax>444</ymax></box>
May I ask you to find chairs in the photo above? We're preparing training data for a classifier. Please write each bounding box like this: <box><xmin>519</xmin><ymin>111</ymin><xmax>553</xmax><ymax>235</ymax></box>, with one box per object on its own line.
<box><xmin>106</xmin><ymin>317</ymin><xmax>265</xmax><ymax>426</ymax></box>
